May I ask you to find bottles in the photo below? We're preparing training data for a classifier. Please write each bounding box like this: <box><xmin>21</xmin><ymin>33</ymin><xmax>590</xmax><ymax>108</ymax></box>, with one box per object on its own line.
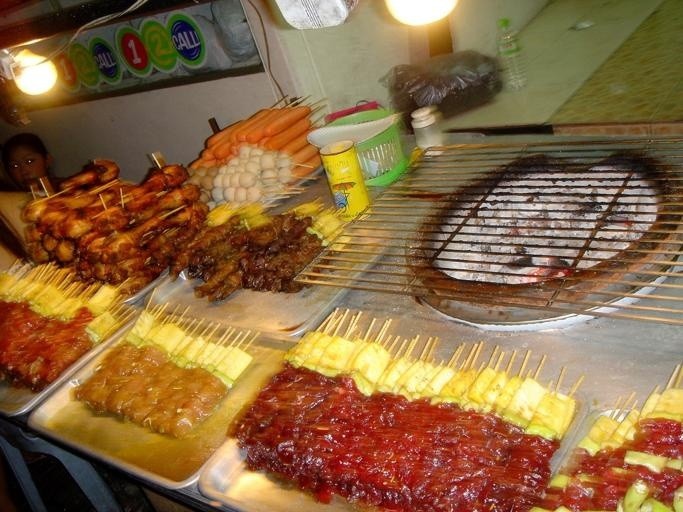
<box><xmin>410</xmin><ymin>107</ymin><xmax>445</xmax><ymax>155</ymax></box>
<box><xmin>495</xmin><ymin>18</ymin><xmax>525</xmax><ymax>90</ymax></box>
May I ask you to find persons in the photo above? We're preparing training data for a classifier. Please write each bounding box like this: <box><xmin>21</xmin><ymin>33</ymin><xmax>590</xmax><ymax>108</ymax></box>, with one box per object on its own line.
<box><xmin>2</xmin><ymin>133</ymin><xmax>61</xmax><ymax>192</ymax></box>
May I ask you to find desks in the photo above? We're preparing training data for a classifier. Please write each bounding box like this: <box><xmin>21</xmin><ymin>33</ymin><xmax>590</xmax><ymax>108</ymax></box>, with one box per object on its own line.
<box><xmin>0</xmin><ymin>116</ymin><xmax>683</xmax><ymax>512</ymax></box>
<box><xmin>440</xmin><ymin>0</ymin><xmax>668</xmax><ymax>131</ymax></box>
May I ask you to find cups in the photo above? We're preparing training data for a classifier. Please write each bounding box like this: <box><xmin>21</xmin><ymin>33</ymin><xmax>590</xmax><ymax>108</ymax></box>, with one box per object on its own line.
<box><xmin>321</xmin><ymin>140</ymin><xmax>370</xmax><ymax>222</ymax></box>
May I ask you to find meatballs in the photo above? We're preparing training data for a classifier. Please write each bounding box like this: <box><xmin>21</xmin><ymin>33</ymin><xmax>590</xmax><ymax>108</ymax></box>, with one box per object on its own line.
<box><xmin>205</xmin><ymin>145</ymin><xmax>293</xmax><ymax>212</ymax></box>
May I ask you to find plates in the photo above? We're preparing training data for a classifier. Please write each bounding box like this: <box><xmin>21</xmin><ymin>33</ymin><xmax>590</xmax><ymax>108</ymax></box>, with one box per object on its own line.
<box><xmin>306</xmin><ymin>122</ymin><xmax>388</xmax><ymax>151</ymax></box>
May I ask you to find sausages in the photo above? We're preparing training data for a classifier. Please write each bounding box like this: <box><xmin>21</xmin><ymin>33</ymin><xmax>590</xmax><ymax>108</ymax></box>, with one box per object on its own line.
<box><xmin>190</xmin><ymin>105</ymin><xmax>322</xmax><ymax>179</ymax></box>
